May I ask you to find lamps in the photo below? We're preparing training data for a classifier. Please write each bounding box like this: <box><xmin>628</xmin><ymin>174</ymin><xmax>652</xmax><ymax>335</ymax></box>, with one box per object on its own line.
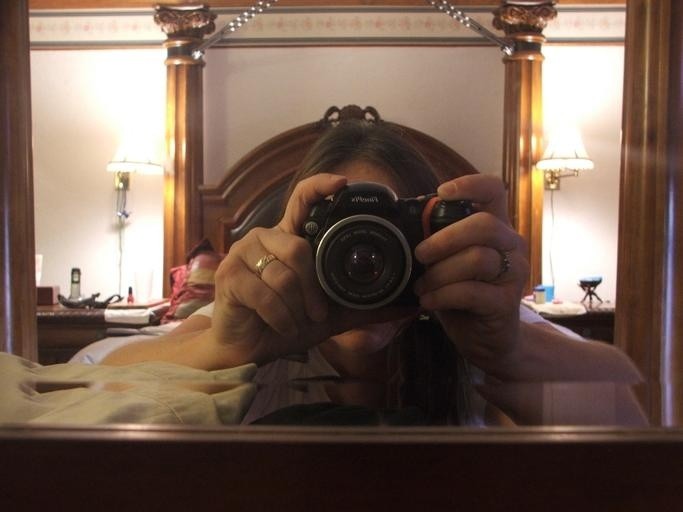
<box><xmin>102</xmin><ymin>136</ymin><xmax>164</xmax><ymax>223</ymax></box>
<box><xmin>536</xmin><ymin>117</ymin><xmax>597</xmax><ymax>192</ymax></box>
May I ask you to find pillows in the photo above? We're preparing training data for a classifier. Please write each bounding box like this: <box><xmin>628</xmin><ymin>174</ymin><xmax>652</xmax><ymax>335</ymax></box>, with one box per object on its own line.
<box><xmin>476</xmin><ymin>370</ymin><xmax>652</xmax><ymax>423</ymax></box>
<box><xmin>172</xmin><ymin>250</ymin><xmax>227</xmax><ymax>301</ymax></box>
<box><xmin>166</xmin><ymin>262</ymin><xmax>216</xmax><ymax>314</ymax></box>
<box><xmin>459</xmin><ymin>317</ymin><xmax>642</xmax><ymax>380</ymax></box>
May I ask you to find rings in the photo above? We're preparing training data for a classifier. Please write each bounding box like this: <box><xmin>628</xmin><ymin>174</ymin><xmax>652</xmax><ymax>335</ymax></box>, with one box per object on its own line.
<box><xmin>251</xmin><ymin>252</ymin><xmax>277</xmax><ymax>280</ymax></box>
<box><xmin>483</xmin><ymin>246</ymin><xmax>515</xmax><ymax>285</ymax></box>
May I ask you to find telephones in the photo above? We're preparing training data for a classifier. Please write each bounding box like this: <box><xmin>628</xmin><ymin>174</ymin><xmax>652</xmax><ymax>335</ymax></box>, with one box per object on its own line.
<box><xmin>67</xmin><ymin>268</ymin><xmax>82</xmax><ymax>302</ymax></box>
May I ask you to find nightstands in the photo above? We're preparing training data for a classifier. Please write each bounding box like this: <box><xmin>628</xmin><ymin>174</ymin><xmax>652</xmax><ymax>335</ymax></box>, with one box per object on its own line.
<box><xmin>520</xmin><ymin>299</ymin><xmax>616</xmax><ymax>343</ymax></box>
<box><xmin>33</xmin><ymin>301</ymin><xmax>160</xmax><ymax>366</ymax></box>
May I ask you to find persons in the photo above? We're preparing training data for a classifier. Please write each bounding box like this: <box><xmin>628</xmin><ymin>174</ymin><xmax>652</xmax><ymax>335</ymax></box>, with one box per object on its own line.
<box><xmin>92</xmin><ymin>121</ymin><xmax>641</xmax><ymax>426</ymax></box>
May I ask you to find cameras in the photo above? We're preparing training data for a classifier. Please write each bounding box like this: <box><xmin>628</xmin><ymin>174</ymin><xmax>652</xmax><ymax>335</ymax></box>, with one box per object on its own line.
<box><xmin>298</xmin><ymin>183</ymin><xmax>473</xmax><ymax>310</ymax></box>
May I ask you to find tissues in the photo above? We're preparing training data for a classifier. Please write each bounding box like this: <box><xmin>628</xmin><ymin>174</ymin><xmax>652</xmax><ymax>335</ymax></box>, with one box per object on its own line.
<box><xmin>35</xmin><ymin>254</ymin><xmax>61</xmax><ymax>306</ymax></box>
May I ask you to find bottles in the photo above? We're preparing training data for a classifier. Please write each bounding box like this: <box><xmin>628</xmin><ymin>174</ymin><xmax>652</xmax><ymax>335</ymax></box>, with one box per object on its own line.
<box><xmin>532</xmin><ymin>285</ymin><xmax>553</xmax><ymax>304</ymax></box>
<box><xmin>126</xmin><ymin>286</ymin><xmax>134</xmax><ymax>303</ymax></box>
<box><xmin>69</xmin><ymin>268</ymin><xmax>80</xmax><ymax>298</ymax></box>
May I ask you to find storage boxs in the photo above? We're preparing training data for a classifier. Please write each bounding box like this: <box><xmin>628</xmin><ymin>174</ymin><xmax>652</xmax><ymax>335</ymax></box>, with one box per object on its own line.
<box><xmin>35</xmin><ymin>285</ymin><xmax>59</xmax><ymax>306</ymax></box>
